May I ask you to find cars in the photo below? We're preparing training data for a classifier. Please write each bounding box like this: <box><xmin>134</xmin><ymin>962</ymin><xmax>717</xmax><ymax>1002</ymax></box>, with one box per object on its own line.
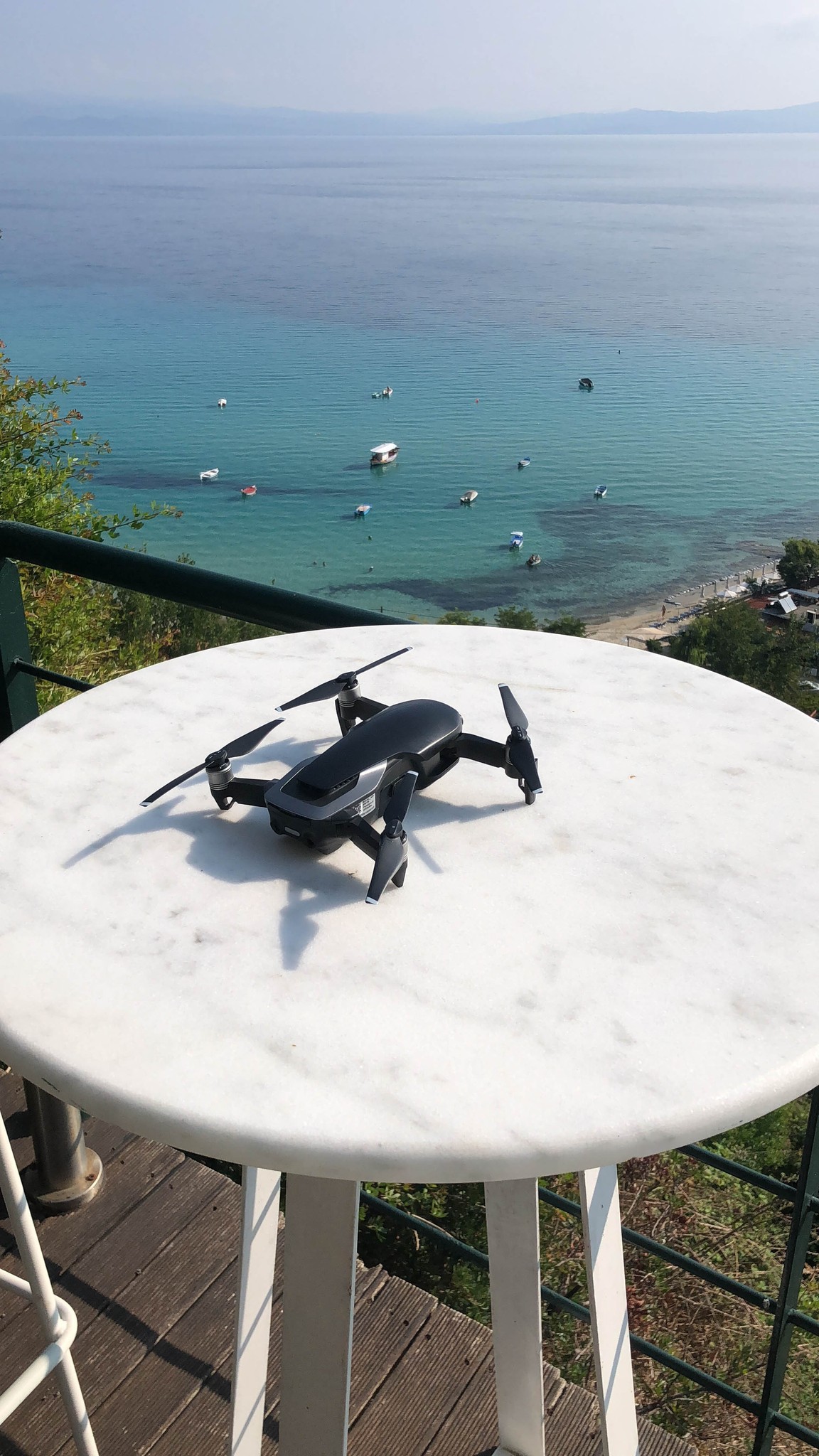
<box><xmin>798</xmin><ymin>681</ymin><xmax>819</xmax><ymax>695</ymax></box>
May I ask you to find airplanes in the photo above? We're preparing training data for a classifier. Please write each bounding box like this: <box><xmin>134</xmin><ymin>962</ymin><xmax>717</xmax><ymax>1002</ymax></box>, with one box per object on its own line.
<box><xmin>139</xmin><ymin>646</ymin><xmax>544</xmax><ymax>905</ymax></box>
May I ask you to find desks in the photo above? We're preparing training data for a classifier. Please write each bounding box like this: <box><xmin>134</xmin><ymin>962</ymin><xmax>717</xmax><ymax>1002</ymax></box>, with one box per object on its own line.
<box><xmin>2</xmin><ymin>623</ymin><xmax>818</xmax><ymax>1454</ymax></box>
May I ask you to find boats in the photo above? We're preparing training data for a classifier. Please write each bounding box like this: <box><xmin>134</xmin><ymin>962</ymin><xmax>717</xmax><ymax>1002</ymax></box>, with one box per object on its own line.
<box><xmin>370</xmin><ymin>443</ymin><xmax>400</xmax><ymax>465</ymax></box>
<box><xmin>460</xmin><ymin>490</ymin><xmax>478</xmax><ymax>503</ymax></box>
<box><xmin>594</xmin><ymin>485</ymin><xmax>607</xmax><ymax>497</ymax></box>
<box><xmin>354</xmin><ymin>503</ymin><xmax>372</xmax><ymax>515</ymax></box>
<box><xmin>517</xmin><ymin>456</ymin><xmax>530</xmax><ymax>466</ymax></box>
<box><xmin>383</xmin><ymin>388</ymin><xmax>393</xmax><ymax>396</ymax></box>
<box><xmin>241</xmin><ymin>484</ymin><xmax>258</xmax><ymax>495</ymax></box>
<box><xmin>199</xmin><ymin>467</ymin><xmax>219</xmax><ymax>480</ymax></box>
<box><xmin>509</xmin><ymin>531</ymin><xmax>524</xmax><ymax>548</ymax></box>
<box><xmin>218</xmin><ymin>397</ymin><xmax>227</xmax><ymax>407</ymax></box>
<box><xmin>578</xmin><ymin>378</ymin><xmax>594</xmax><ymax>388</ymax></box>
<box><xmin>527</xmin><ymin>555</ymin><xmax>541</xmax><ymax>566</ymax></box>
<box><xmin>371</xmin><ymin>392</ymin><xmax>383</xmax><ymax>398</ymax></box>
